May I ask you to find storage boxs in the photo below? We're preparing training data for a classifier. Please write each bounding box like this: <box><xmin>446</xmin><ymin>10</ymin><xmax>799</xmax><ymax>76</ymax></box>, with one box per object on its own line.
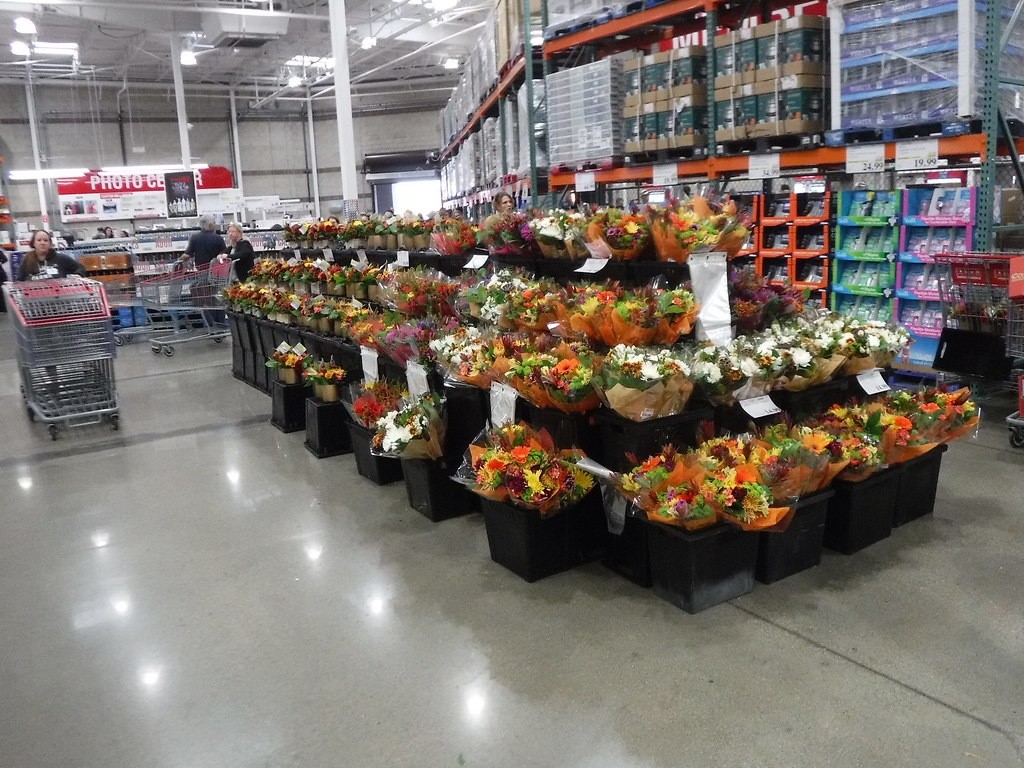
<box><xmin>546</xmin><ymin>15</ymin><xmax>831</xmax><ymax>168</ymax></box>
<box><xmin>81</xmin><ymin>254</ymin><xmax>134</xmax><ymax>290</ymax></box>
<box><xmin>228</xmin><ymin>235</ymin><xmax>947</xmax><ymax>614</ymax></box>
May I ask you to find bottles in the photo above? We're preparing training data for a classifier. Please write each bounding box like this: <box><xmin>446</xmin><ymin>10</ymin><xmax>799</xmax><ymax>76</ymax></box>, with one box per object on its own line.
<box><xmin>177</xmin><ymin>198</ymin><xmax>182</xmax><ymax>211</ymax></box>
<box><xmin>168</xmin><ymin>201</ymin><xmax>173</xmax><ymax>211</ymax></box>
<box><xmin>190</xmin><ymin>199</ymin><xmax>195</xmax><ymax>210</ymax></box>
<box><xmin>182</xmin><ymin>197</ymin><xmax>186</xmax><ymax>211</ymax></box>
<box><xmin>186</xmin><ymin>198</ymin><xmax>190</xmax><ymax>210</ymax></box>
<box><xmin>173</xmin><ymin>200</ymin><xmax>177</xmax><ymax>212</ymax></box>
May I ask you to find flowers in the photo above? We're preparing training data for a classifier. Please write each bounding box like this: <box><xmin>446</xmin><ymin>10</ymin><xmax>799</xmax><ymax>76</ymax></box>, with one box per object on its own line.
<box><xmin>217</xmin><ymin>193</ymin><xmax>983</xmax><ymax>534</ymax></box>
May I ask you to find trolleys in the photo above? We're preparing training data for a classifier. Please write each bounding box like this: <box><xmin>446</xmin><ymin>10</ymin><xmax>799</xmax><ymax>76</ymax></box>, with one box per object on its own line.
<box><xmin>928</xmin><ymin>248</ymin><xmax>1024</xmax><ymax>451</ymax></box>
<box><xmin>139</xmin><ymin>256</ymin><xmax>241</xmax><ymax>357</ymax></box>
<box><xmin>103</xmin><ymin>257</ymin><xmax>195</xmax><ymax>346</ymax></box>
<box><xmin>0</xmin><ymin>275</ymin><xmax>121</xmax><ymax>441</ymax></box>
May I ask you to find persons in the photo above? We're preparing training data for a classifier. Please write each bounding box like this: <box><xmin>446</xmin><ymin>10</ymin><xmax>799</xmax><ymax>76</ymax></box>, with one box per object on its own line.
<box><xmin>484</xmin><ymin>191</ymin><xmax>515</xmax><ymax>222</ymax></box>
<box><xmin>177</xmin><ymin>211</ymin><xmax>227</xmax><ymax>338</ymax></box>
<box><xmin>15</xmin><ymin>229</ymin><xmax>88</xmax><ymax>394</ymax></box>
<box><xmin>63</xmin><ymin>200</ymin><xmax>97</xmax><ymax>215</ymax></box>
<box><xmin>327</xmin><ymin>208</ymin><xmax>462</xmax><ymax>225</ymax></box>
<box><xmin>92</xmin><ymin>226</ymin><xmax>129</xmax><ymax>239</ymax></box>
<box><xmin>0</xmin><ymin>250</ymin><xmax>9</xmax><ymax>313</ymax></box>
<box><xmin>217</xmin><ymin>221</ymin><xmax>256</xmax><ymax>285</ymax></box>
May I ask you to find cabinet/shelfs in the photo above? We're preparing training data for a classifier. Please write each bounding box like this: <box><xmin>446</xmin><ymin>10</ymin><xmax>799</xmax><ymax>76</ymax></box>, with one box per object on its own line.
<box><xmin>438</xmin><ymin>0</ymin><xmax>1024</xmax><ymax>402</ymax></box>
